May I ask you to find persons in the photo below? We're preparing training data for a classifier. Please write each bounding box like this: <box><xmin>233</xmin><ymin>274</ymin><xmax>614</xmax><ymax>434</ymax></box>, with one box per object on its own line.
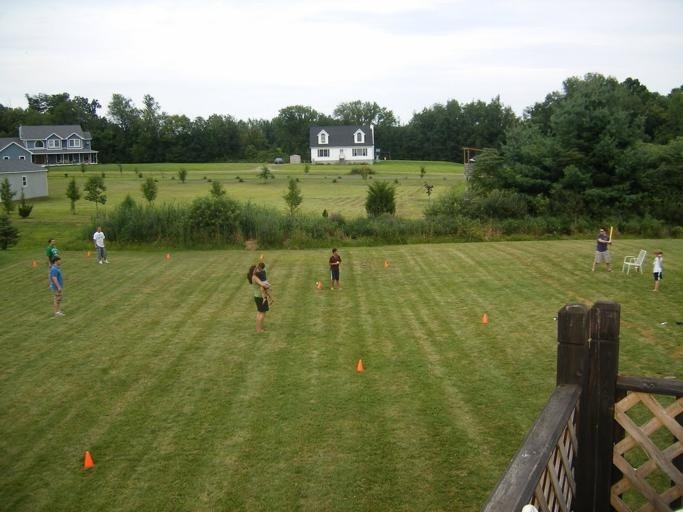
<box><xmin>651</xmin><ymin>249</ymin><xmax>664</xmax><ymax>291</ymax></box>
<box><xmin>328</xmin><ymin>248</ymin><xmax>342</xmax><ymax>291</ymax></box>
<box><xmin>93</xmin><ymin>226</ymin><xmax>110</xmax><ymax>264</ymax></box>
<box><xmin>49</xmin><ymin>255</ymin><xmax>66</xmax><ymax>316</ymax></box>
<box><xmin>592</xmin><ymin>228</ymin><xmax>613</xmax><ymax>273</ymax></box>
<box><xmin>255</xmin><ymin>263</ymin><xmax>274</xmax><ymax>306</ymax></box>
<box><xmin>247</xmin><ymin>265</ymin><xmax>269</xmax><ymax>333</ymax></box>
<box><xmin>45</xmin><ymin>239</ymin><xmax>59</xmax><ymax>268</ymax></box>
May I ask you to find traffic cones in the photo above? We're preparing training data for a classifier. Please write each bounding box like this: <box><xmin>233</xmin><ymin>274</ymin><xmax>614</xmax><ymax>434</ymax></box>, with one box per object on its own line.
<box><xmin>83</xmin><ymin>450</ymin><xmax>95</xmax><ymax>468</ymax></box>
<box><xmin>383</xmin><ymin>260</ymin><xmax>389</xmax><ymax>268</ymax></box>
<box><xmin>316</xmin><ymin>280</ymin><xmax>324</xmax><ymax>290</ymax></box>
<box><xmin>481</xmin><ymin>311</ymin><xmax>489</xmax><ymax>324</ymax></box>
<box><xmin>356</xmin><ymin>357</ymin><xmax>364</xmax><ymax>372</ymax></box>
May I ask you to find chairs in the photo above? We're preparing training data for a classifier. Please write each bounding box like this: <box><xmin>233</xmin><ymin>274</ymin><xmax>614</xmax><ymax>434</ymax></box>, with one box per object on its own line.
<box><xmin>621</xmin><ymin>248</ymin><xmax>646</xmax><ymax>276</ymax></box>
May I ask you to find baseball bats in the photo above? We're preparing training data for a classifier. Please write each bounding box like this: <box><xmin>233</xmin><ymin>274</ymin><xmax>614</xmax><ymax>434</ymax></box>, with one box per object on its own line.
<box><xmin>609</xmin><ymin>226</ymin><xmax>613</xmax><ymax>241</ymax></box>
